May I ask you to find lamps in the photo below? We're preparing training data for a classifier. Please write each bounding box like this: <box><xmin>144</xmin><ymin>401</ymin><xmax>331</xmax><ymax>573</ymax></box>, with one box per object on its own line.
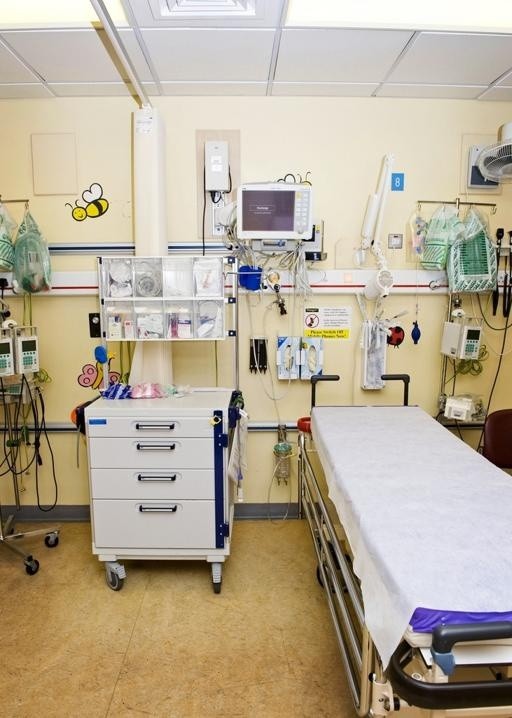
<box><xmin>361</xmin><ymin>153</ymin><xmax>398</xmax><ymax>301</ymax></box>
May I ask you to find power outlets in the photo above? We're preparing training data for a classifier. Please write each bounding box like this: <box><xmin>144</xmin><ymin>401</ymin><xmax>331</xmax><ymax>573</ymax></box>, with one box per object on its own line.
<box><xmin>497</xmin><ymin>269</ymin><xmax>511</xmax><ymax>286</ymax></box>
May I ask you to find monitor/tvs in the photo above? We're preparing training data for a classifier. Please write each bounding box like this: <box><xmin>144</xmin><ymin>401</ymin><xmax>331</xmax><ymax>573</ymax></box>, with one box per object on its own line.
<box><xmin>236</xmin><ymin>185</ymin><xmax>312</xmax><ymax>240</ymax></box>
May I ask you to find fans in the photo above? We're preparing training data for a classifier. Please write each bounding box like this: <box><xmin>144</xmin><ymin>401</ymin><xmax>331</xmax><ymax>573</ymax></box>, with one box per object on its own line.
<box><xmin>461</xmin><ymin>122</ymin><xmax>511</xmax><ymax>192</ymax></box>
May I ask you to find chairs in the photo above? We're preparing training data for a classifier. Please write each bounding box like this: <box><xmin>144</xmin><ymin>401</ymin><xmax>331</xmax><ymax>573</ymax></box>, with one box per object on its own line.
<box><xmin>473</xmin><ymin>407</ymin><xmax>512</xmax><ymax>467</ymax></box>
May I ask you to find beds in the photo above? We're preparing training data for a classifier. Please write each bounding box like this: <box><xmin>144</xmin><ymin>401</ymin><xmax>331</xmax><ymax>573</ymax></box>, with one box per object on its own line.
<box><xmin>291</xmin><ymin>406</ymin><xmax>512</xmax><ymax>718</ymax></box>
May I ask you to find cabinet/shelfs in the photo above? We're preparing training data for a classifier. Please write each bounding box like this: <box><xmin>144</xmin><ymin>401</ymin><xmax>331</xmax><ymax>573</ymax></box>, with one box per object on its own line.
<box><xmin>81</xmin><ymin>383</ymin><xmax>242</xmax><ymax>593</ymax></box>
<box><xmin>96</xmin><ymin>254</ymin><xmax>242</xmax><ymax>344</ymax></box>
